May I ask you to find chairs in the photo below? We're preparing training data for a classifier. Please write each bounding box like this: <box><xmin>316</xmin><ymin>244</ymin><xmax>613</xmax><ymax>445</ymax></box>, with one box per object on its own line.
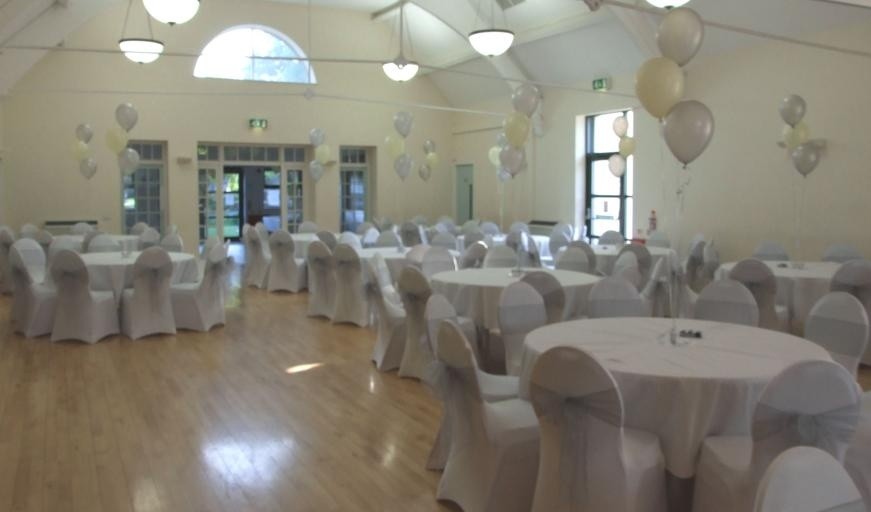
<box><xmin>1</xmin><ymin>222</ymin><xmax>233</xmax><ymax>347</ymax></box>
